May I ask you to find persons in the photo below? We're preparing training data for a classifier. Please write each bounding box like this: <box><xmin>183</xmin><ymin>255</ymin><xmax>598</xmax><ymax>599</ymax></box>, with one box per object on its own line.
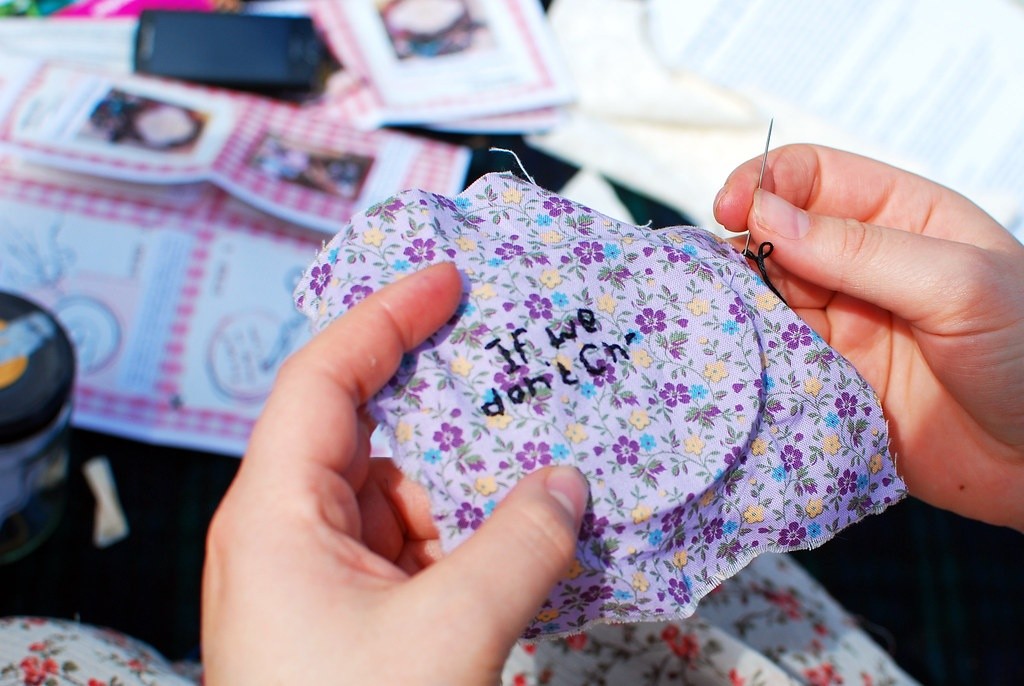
<box><xmin>201</xmin><ymin>143</ymin><xmax>1024</xmax><ymax>686</ymax></box>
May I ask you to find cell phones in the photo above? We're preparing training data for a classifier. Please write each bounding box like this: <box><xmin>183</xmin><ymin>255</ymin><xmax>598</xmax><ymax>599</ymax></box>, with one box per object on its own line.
<box><xmin>132</xmin><ymin>9</ymin><xmax>320</xmax><ymax>98</ymax></box>
